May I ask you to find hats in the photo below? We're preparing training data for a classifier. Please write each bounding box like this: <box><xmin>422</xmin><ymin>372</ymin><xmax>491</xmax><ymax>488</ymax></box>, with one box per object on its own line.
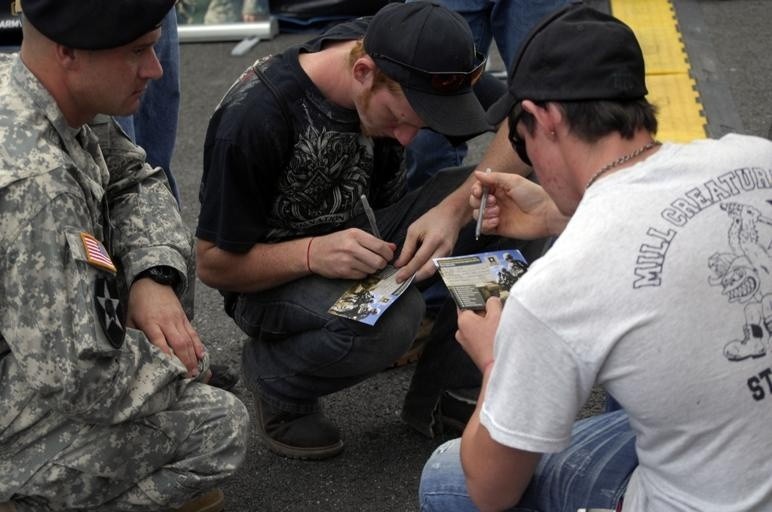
<box><xmin>19</xmin><ymin>0</ymin><xmax>177</xmax><ymax>51</ymax></box>
<box><xmin>363</xmin><ymin>1</ymin><xmax>497</xmax><ymax>137</ymax></box>
<box><xmin>485</xmin><ymin>0</ymin><xmax>647</xmax><ymax>126</ymax></box>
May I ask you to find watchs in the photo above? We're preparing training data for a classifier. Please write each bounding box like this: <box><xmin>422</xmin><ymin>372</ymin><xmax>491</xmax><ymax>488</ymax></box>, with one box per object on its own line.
<box><xmin>131</xmin><ymin>267</ymin><xmax>185</xmax><ymax>295</ymax></box>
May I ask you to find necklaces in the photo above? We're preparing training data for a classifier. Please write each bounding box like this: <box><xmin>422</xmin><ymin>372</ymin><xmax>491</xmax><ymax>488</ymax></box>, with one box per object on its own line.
<box><xmin>584</xmin><ymin>141</ymin><xmax>665</xmax><ymax>188</ymax></box>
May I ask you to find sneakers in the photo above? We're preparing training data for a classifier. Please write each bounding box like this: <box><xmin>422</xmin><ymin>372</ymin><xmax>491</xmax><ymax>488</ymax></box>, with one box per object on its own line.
<box><xmin>240</xmin><ymin>334</ymin><xmax>346</xmax><ymax>462</ymax></box>
<box><xmin>410</xmin><ymin>393</ymin><xmax>477</xmax><ymax>439</ymax></box>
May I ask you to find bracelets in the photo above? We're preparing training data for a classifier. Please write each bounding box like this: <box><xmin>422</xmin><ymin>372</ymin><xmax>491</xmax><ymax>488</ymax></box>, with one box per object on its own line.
<box><xmin>307</xmin><ymin>235</ymin><xmax>321</xmax><ymax>276</ymax></box>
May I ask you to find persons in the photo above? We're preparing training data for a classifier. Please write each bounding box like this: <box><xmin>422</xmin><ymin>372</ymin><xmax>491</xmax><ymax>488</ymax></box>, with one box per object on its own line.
<box><xmin>374</xmin><ymin>0</ymin><xmax>578</xmax><ymax>372</ymax></box>
<box><xmin>417</xmin><ymin>4</ymin><xmax>772</xmax><ymax>512</ymax></box>
<box><xmin>196</xmin><ymin>2</ymin><xmax>559</xmax><ymax>461</ymax></box>
<box><xmin>109</xmin><ymin>1</ymin><xmax>239</xmax><ymax>391</ymax></box>
<box><xmin>0</xmin><ymin>0</ymin><xmax>251</xmax><ymax>511</ymax></box>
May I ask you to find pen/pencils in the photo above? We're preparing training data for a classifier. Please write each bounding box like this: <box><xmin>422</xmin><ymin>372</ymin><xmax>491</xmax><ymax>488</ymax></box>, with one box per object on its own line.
<box><xmin>360</xmin><ymin>194</ymin><xmax>380</xmax><ymax>239</ymax></box>
<box><xmin>475</xmin><ymin>168</ymin><xmax>491</xmax><ymax>240</ymax></box>
